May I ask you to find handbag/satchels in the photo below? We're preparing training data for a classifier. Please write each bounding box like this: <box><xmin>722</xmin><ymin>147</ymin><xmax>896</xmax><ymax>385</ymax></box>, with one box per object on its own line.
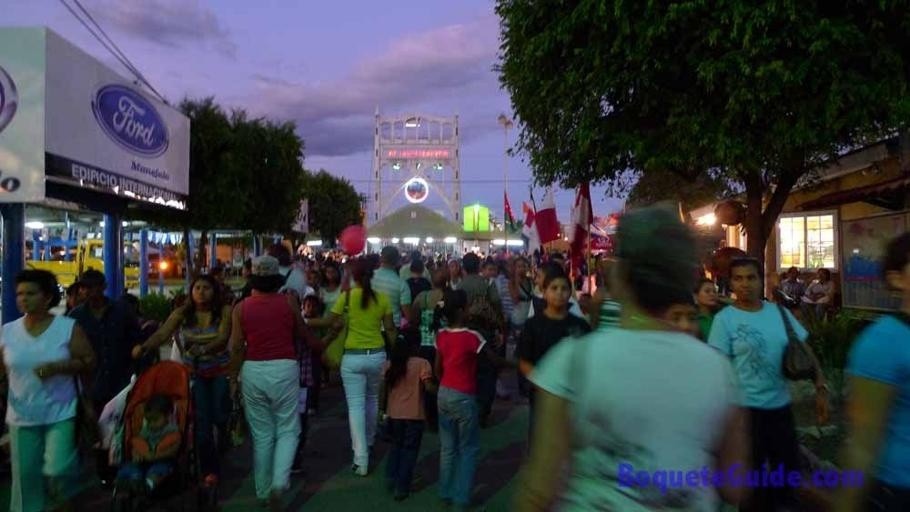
<box><xmin>69</xmin><ymin>353</ymin><xmax>103</xmax><ymax>444</ymax></box>
<box><xmin>777</xmin><ymin>302</ymin><xmax>816</xmax><ymax>381</ymax></box>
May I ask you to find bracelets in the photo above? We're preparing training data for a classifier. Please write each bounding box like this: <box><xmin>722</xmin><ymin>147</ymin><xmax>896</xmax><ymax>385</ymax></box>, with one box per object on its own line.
<box><xmin>814</xmin><ymin>382</ymin><xmax>826</xmax><ymax>392</ymax></box>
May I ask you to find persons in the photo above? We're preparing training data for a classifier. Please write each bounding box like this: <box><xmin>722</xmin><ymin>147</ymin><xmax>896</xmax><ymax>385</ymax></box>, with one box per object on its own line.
<box><xmin>0</xmin><ymin>269</ymin><xmax>96</xmax><ymax>512</ymax></box>
<box><xmin>229</xmin><ymin>254</ymin><xmax>344</xmax><ymax>502</ymax></box>
<box><xmin>837</xmin><ymin>233</ymin><xmax>910</xmax><ymax>511</ymax></box>
<box><xmin>518</xmin><ymin>271</ymin><xmax>590</xmax><ymax>382</ymax></box>
<box><xmin>1</xmin><ymin>238</ymin><xmax>850</xmax><ymax>512</ymax></box>
<box><xmin>705</xmin><ymin>252</ymin><xmax>833</xmax><ymax>491</ymax></box>
<box><xmin>431</xmin><ymin>286</ymin><xmax>512</xmax><ymax>511</ymax></box>
<box><xmin>509</xmin><ymin>204</ymin><xmax>749</xmax><ymax>510</ymax></box>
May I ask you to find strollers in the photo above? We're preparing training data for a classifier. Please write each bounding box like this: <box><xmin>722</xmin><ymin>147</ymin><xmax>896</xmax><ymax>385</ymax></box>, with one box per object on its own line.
<box><xmin>98</xmin><ymin>342</ymin><xmax>204</xmax><ymax>509</ymax></box>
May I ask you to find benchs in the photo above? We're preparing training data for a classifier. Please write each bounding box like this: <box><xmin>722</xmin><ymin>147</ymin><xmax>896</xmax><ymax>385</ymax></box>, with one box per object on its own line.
<box><xmin>778</xmin><ymin>271</ymin><xmax>841</xmax><ymax>324</ymax></box>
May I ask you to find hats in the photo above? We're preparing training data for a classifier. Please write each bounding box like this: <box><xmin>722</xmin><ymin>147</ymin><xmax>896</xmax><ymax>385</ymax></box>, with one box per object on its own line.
<box><xmin>252</xmin><ymin>256</ymin><xmax>279</xmax><ymax>275</ymax></box>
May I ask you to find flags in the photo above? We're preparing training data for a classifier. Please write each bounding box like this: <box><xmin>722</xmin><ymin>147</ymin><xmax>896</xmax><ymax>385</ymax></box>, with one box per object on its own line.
<box><xmin>534</xmin><ymin>185</ymin><xmax>560</xmax><ymax>243</ymax></box>
<box><xmin>501</xmin><ymin>189</ymin><xmax>520</xmax><ymax>238</ymax></box>
<box><xmin>520</xmin><ymin>195</ymin><xmax>543</xmax><ymax>266</ymax></box>
<box><xmin>562</xmin><ymin>181</ymin><xmax>593</xmax><ymax>281</ymax></box>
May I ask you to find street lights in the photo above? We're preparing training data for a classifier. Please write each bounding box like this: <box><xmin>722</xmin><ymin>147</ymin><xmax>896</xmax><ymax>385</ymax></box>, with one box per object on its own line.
<box><xmin>496</xmin><ymin>114</ymin><xmax>514</xmax><ymax>260</ymax></box>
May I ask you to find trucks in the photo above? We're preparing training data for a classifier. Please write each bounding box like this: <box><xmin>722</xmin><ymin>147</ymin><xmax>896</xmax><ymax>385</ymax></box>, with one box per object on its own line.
<box><xmin>28</xmin><ymin>240</ymin><xmax>143</xmax><ymax>297</ymax></box>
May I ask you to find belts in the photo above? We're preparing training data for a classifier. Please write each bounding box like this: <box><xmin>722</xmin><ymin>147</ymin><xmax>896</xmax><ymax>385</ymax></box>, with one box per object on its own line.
<box><xmin>344</xmin><ymin>348</ymin><xmax>383</xmax><ymax>355</ymax></box>
